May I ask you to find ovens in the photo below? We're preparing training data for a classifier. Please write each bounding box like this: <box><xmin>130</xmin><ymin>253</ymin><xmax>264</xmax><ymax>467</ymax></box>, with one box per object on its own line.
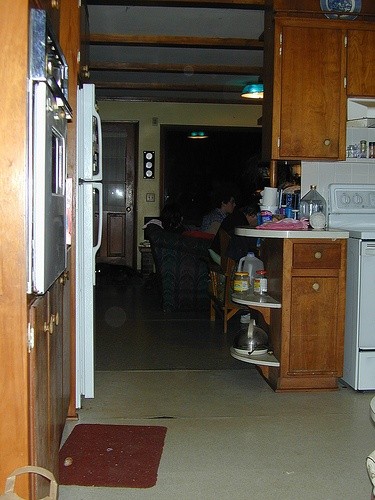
<box><xmin>340</xmin><ymin>238</ymin><xmax>375</xmax><ymax>391</ymax></box>
<box><xmin>27</xmin><ymin>7</ymin><xmax>67</xmax><ymax>297</ymax></box>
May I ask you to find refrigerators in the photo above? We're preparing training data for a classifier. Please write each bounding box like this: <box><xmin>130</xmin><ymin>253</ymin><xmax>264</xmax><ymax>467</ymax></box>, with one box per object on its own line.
<box><xmin>76</xmin><ymin>84</ymin><xmax>105</xmax><ymax>408</ymax></box>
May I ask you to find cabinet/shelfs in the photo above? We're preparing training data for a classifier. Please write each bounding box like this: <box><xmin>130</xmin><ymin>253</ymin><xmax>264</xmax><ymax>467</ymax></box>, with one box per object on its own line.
<box><xmin>27</xmin><ymin>268</ymin><xmax>70</xmax><ymax>500</ymax></box>
<box><xmin>271</xmin><ymin>18</ymin><xmax>347</xmax><ymax>162</ymax></box>
<box><xmin>347</xmin><ymin>20</ymin><xmax>375</xmax><ymax>98</ymax></box>
<box><xmin>229</xmin><ymin>227</ymin><xmax>350</xmax><ymax>392</ymax></box>
<box><xmin>33</xmin><ymin>0</ymin><xmax>91</xmax><ymax>84</ymax></box>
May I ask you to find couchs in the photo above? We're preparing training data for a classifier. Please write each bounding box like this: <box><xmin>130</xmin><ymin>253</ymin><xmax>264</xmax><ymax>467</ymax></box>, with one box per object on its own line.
<box><xmin>144</xmin><ymin>219</ymin><xmax>222</xmax><ymax>314</ymax></box>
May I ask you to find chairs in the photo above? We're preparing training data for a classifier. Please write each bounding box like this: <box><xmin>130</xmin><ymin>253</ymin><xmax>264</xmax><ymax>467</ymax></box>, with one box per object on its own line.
<box><xmin>206</xmin><ymin>256</ymin><xmax>243</xmax><ymax>334</ymax></box>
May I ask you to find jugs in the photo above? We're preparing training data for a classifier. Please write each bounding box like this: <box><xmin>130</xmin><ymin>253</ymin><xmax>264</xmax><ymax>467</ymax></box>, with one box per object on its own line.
<box><xmin>235</xmin><ymin>319</ymin><xmax>268</xmax><ymax>355</ymax></box>
<box><xmin>237</xmin><ymin>251</ymin><xmax>264</xmax><ymax>294</ymax></box>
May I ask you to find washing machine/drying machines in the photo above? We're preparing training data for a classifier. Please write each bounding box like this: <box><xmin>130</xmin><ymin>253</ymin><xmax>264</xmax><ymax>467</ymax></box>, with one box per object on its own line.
<box><xmin>328</xmin><ymin>182</ymin><xmax>375</xmax><ymax>392</ymax></box>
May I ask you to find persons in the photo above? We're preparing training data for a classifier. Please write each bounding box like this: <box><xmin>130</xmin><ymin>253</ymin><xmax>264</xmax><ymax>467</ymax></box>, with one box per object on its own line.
<box><xmin>199</xmin><ymin>185</ymin><xmax>239</xmax><ymax>234</ymax></box>
<box><xmin>215</xmin><ymin>206</ymin><xmax>263</xmax><ymax>269</ymax></box>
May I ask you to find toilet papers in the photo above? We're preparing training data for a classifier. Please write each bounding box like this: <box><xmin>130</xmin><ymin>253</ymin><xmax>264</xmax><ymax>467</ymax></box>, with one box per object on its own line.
<box><xmin>260</xmin><ymin>186</ymin><xmax>278</xmax><ymax>207</ymax></box>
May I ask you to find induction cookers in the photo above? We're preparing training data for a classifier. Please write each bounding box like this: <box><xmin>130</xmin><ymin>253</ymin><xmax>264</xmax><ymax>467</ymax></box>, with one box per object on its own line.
<box><xmin>326</xmin><ymin>183</ymin><xmax>375</xmax><ymax>240</ymax></box>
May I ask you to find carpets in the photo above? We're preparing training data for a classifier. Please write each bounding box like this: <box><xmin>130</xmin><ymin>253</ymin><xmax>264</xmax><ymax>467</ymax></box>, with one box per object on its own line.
<box><xmin>59</xmin><ymin>424</ymin><xmax>168</xmax><ymax>488</ymax></box>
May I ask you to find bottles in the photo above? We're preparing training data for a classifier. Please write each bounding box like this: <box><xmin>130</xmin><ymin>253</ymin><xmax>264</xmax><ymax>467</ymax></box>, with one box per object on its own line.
<box><xmin>300</xmin><ymin>185</ymin><xmax>326</xmax><ymax>219</ymax></box>
<box><xmin>348</xmin><ymin>140</ymin><xmax>375</xmax><ymax>158</ymax></box>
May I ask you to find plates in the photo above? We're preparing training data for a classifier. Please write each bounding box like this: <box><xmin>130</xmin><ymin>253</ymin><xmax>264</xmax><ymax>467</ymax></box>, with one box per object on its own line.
<box><xmin>320</xmin><ymin>0</ymin><xmax>361</xmax><ymax>20</ymax></box>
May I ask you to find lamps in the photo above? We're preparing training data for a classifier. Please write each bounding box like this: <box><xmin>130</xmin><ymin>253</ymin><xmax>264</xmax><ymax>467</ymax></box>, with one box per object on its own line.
<box><xmin>240</xmin><ymin>81</ymin><xmax>264</xmax><ymax>99</ymax></box>
